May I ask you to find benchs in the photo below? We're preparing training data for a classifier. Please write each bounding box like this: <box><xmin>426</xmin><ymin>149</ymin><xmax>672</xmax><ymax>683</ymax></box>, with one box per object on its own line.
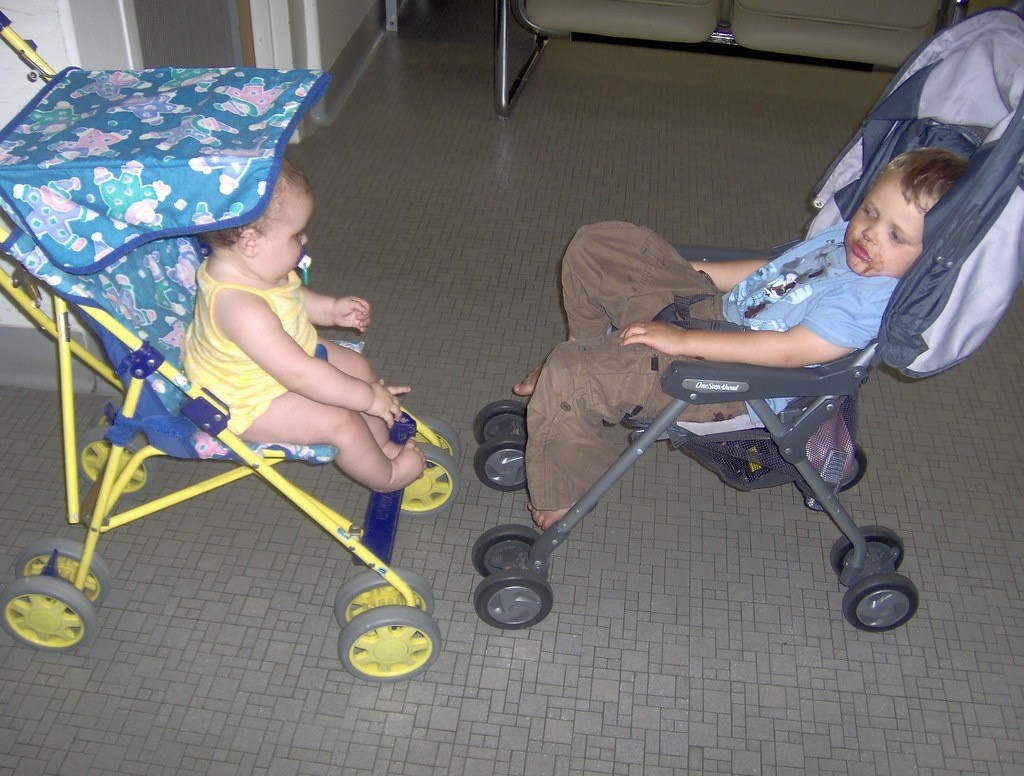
<box><xmin>492</xmin><ymin>0</ymin><xmax>969</xmax><ymax>122</ymax></box>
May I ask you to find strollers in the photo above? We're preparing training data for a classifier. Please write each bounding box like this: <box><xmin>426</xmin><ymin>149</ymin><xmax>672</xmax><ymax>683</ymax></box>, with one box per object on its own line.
<box><xmin>0</xmin><ymin>13</ymin><xmax>465</xmax><ymax>685</ymax></box>
<box><xmin>470</xmin><ymin>1</ymin><xmax>1024</xmax><ymax>634</ymax></box>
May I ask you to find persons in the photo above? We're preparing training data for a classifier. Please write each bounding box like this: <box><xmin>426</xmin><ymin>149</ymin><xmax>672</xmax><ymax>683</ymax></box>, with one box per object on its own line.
<box><xmin>183</xmin><ymin>149</ymin><xmax>426</xmax><ymax>494</ymax></box>
<box><xmin>513</xmin><ymin>146</ymin><xmax>968</xmax><ymax>532</ymax></box>
